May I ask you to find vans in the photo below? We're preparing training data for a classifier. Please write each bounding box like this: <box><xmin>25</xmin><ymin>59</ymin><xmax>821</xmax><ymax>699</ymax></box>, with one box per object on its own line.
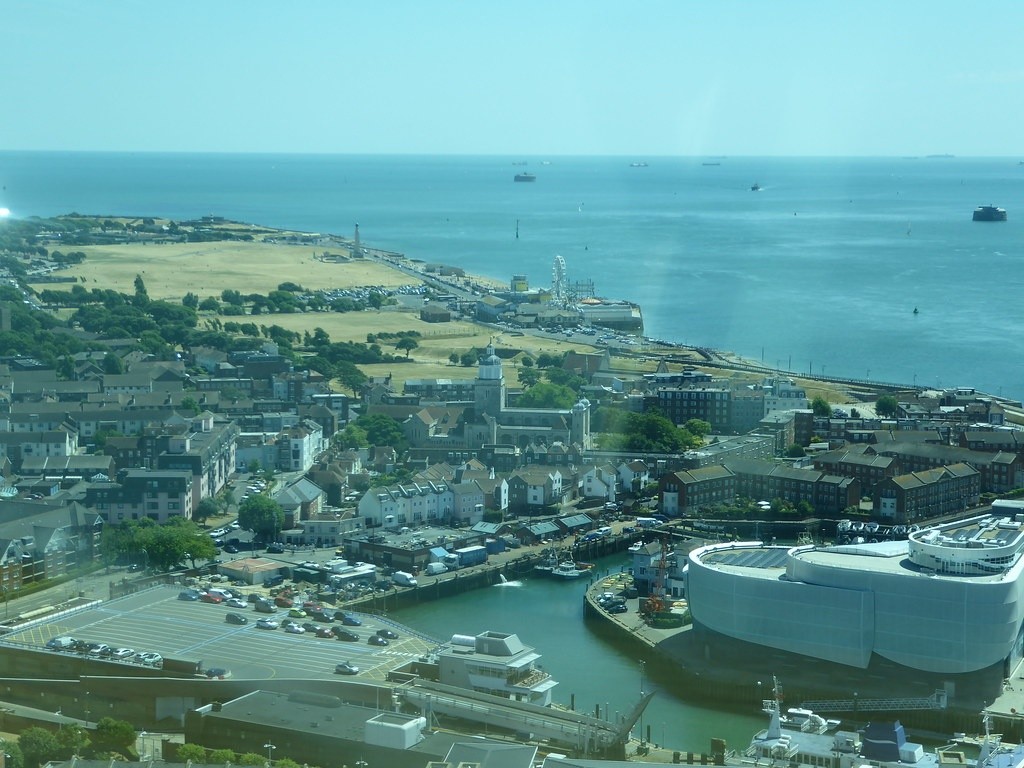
<box><xmin>652</xmin><ymin>514</ymin><xmax>670</xmax><ymax>522</ymax></box>
<box><xmin>596</xmin><ymin>527</ymin><xmax>612</xmax><ymax>536</ymax></box>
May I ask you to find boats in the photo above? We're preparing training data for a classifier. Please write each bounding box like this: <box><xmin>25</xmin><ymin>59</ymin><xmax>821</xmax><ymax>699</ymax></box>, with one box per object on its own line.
<box><xmin>745</xmin><ymin>673</ymin><xmax>1024</xmax><ymax>768</ymax></box>
<box><xmin>974</xmin><ymin>205</ymin><xmax>1007</xmax><ymax>222</ymax></box>
<box><xmin>838</xmin><ymin>521</ymin><xmax>879</xmax><ymax>533</ymax></box>
<box><xmin>532</xmin><ymin>541</ymin><xmax>596</xmax><ymax>581</ymax></box>
<box><xmin>515</xmin><ymin>174</ymin><xmax>536</xmax><ymax>182</ymax></box>
<box><xmin>892</xmin><ymin>525</ymin><xmax>933</xmax><ymax>534</ymax></box>
<box><xmin>628</xmin><ymin>541</ymin><xmax>647</xmax><ymax>555</ymax></box>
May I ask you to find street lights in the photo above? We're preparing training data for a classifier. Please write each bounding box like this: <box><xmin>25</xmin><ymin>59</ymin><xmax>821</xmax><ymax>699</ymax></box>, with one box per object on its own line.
<box><xmin>639</xmin><ymin>659</ymin><xmax>646</xmax><ymax>745</ymax></box>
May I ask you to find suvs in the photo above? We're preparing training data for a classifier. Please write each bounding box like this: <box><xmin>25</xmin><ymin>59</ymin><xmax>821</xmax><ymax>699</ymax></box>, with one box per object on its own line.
<box><xmin>256</xmin><ymin>617</ymin><xmax>279</xmax><ymax>630</ymax></box>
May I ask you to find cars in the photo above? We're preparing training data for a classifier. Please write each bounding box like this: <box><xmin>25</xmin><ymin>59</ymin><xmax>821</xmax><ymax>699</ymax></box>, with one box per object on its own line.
<box><xmin>282</xmin><ymin>619</ymin><xmax>360</xmax><ymax>641</ymax></box>
<box><xmin>267</xmin><ymin>544</ymin><xmax>285</xmax><ymax>554</ymax></box>
<box><xmin>225</xmin><ymin>612</ymin><xmax>248</xmax><ymax>625</ymax></box>
<box><xmin>177</xmin><ymin>587</ymin><xmax>248</xmax><ymax>608</ymax></box>
<box><xmin>335</xmin><ymin>660</ymin><xmax>358</xmax><ymax>674</ymax></box>
<box><xmin>241</xmin><ymin>468</ymin><xmax>282</xmax><ymax>502</ymax></box>
<box><xmin>248</xmin><ymin>593</ymin><xmax>278</xmax><ymax>612</ymax></box>
<box><xmin>582</xmin><ymin>532</ymin><xmax>604</xmax><ymax>543</ymax></box>
<box><xmin>597</xmin><ymin>592</ymin><xmax>628</xmax><ymax>613</ymax></box>
<box><xmin>368</xmin><ymin>629</ymin><xmax>400</xmax><ymax>646</ymax></box>
<box><xmin>46</xmin><ymin>637</ymin><xmax>163</xmax><ymax>667</ymax></box>
<box><xmin>210</xmin><ymin>521</ymin><xmax>240</xmax><ymax>553</ymax></box>
<box><xmin>289</xmin><ymin>601</ymin><xmax>362</xmax><ymax>626</ymax></box>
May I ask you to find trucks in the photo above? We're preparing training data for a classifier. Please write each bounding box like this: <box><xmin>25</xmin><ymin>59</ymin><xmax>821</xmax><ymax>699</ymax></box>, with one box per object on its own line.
<box><xmin>427</xmin><ymin>545</ymin><xmax>487</xmax><ymax>575</ymax></box>
<box><xmin>394</xmin><ymin>571</ymin><xmax>417</xmax><ymax>586</ymax></box>
